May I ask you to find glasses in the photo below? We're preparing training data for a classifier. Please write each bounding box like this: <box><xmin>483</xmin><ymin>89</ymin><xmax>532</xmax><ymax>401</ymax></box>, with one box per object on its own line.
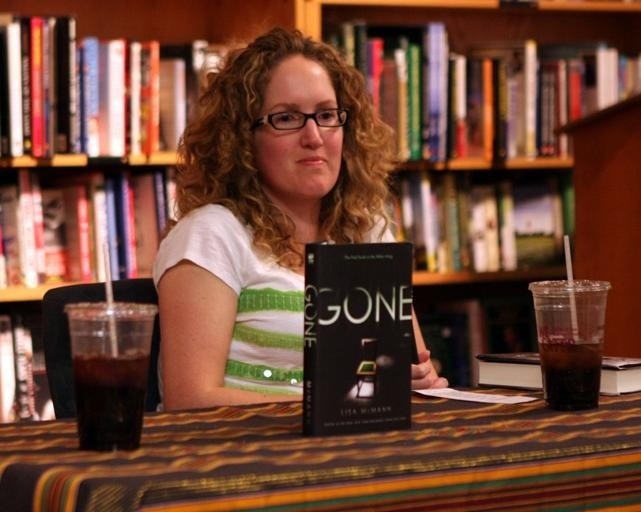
<box><xmin>248</xmin><ymin>106</ymin><xmax>354</xmax><ymax>131</ymax></box>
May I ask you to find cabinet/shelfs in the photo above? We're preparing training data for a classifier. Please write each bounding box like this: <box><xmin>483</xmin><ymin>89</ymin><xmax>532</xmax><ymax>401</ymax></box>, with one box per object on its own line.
<box><xmin>2</xmin><ymin>0</ymin><xmax>305</xmax><ymax>421</ymax></box>
<box><xmin>306</xmin><ymin>1</ymin><xmax>639</xmax><ymax>380</ymax></box>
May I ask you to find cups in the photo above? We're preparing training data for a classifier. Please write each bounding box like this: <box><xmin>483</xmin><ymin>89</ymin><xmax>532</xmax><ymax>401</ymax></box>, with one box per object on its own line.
<box><xmin>61</xmin><ymin>301</ymin><xmax>158</xmax><ymax>449</ymax></box>
<box><xmin>529</xmin><ymin>278</ymin><xmax>612</xmax><ymax>412</ymax></box>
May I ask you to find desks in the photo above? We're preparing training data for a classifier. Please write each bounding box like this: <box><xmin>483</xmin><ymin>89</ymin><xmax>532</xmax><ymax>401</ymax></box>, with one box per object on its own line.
<box><xmin>1</xmin><ymin>404</ymin><xmax>640</xmax><ymax>510</ymax></box>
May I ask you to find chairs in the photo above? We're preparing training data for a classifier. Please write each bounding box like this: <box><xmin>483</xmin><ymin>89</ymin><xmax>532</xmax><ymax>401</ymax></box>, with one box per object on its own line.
<box><xmin>37</xmin><ymin>275</ymin><xmax>168</xmax><ymax>419</ymax></box>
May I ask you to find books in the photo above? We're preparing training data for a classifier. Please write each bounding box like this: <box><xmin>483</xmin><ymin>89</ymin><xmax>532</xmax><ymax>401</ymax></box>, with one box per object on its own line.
<box><xmin>300</xmin><ymin>240</ymin><xmax>413</xmax><ymax>436</ymax></box>
